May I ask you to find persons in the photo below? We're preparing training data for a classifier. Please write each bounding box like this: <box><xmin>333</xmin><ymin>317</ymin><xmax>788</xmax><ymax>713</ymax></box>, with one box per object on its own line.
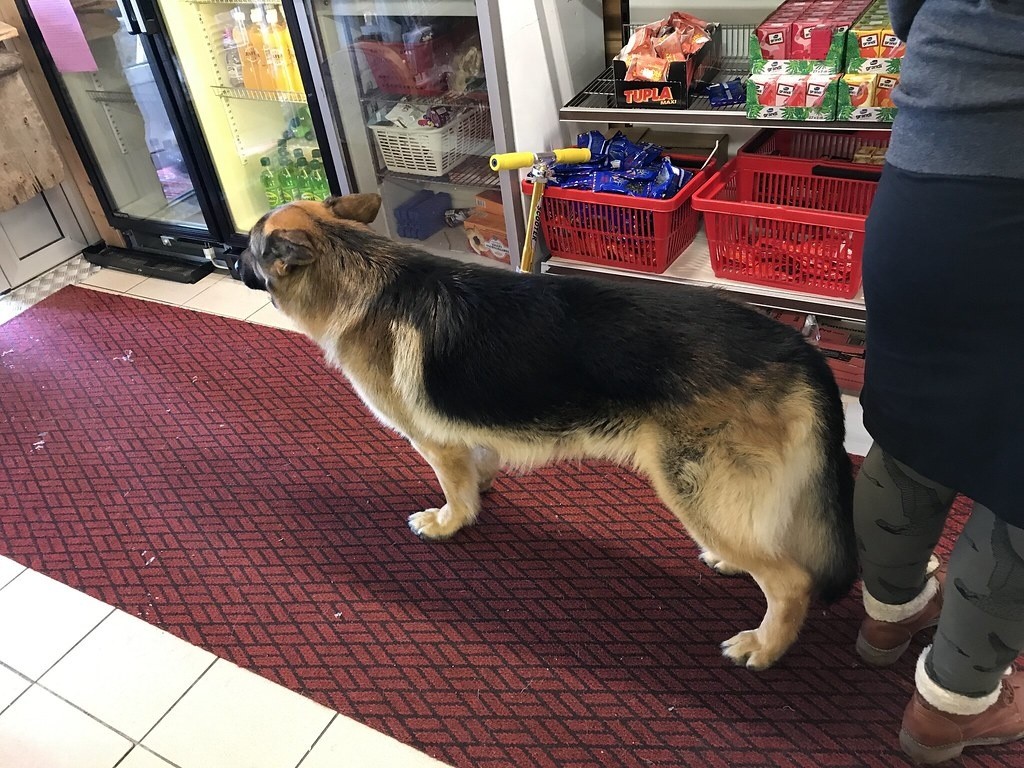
<box><xmin>848</xmin><ymin>0</ymin><xmax>1024</xmax><ymax>763</ymax></box>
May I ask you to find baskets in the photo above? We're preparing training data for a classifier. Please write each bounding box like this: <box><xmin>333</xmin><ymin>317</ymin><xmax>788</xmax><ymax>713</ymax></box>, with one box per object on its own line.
<box><xmin>736</xmin><ymin>127</ymin><xmax>891</xmax><ymax>168</ymax></box>
<box><xmin>522</xmin><ymin>145</ymin><xmax>717</xmax><ymax>275</ymax></box>
<box><xmin>354</xmin><ymin>23</ymin><xmax>469</xmax><ymax>94</ymax></box>
<box><xmin>367</xmin><ymin>95</ymin><xmax>492</xmax><ymax>176</ymax></box>
<box><xmin>691</xmin><ymin>154</ymin><xmax>885</xmax><ymax>300</ymax></box>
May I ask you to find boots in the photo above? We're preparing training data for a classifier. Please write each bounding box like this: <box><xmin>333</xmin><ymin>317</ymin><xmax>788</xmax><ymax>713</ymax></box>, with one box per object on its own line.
<box><xmin>855</xmin><ymin>551</ymin><xmax>948</xmax><ymax>667</ymax></box>
<box><xmin>899</xmin><ymin>643</ymin><xmax>1024</xmax><ymax>764</ymax></box>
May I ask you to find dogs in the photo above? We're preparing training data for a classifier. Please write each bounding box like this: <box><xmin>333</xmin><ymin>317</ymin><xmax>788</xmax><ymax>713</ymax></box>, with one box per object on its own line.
<box><xmin>233</xmin><ymin>189</ymin><xmax>861</xmax><ymax>673</ymax></box>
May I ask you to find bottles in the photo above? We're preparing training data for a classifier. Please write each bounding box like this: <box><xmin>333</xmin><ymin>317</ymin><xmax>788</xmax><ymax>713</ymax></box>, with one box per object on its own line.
<box><xmin>223</xmin><ymin>4</ymin><xmax>304</xmax><ymax>99</ymax></box>
<box><xmin>258</xmin><ymin>105</ymin><xmax>331</xmax><ymax>209</ymax></box>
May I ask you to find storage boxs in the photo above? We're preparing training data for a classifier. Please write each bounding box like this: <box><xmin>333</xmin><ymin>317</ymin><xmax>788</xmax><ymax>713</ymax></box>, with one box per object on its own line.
<box><xmin>463</xmin><ymin>190</ymin><xmax>511</xmax><ymax>265</ymax></box>
<box><xmin>611</xmin><ymin>1</ymin><xmax>906</xmax><ymax>123</ymax></box>
<box><xmin>605</xmin><ymin>127</ymin><xmax>729</xmax><ymax>170</ymax></box>
